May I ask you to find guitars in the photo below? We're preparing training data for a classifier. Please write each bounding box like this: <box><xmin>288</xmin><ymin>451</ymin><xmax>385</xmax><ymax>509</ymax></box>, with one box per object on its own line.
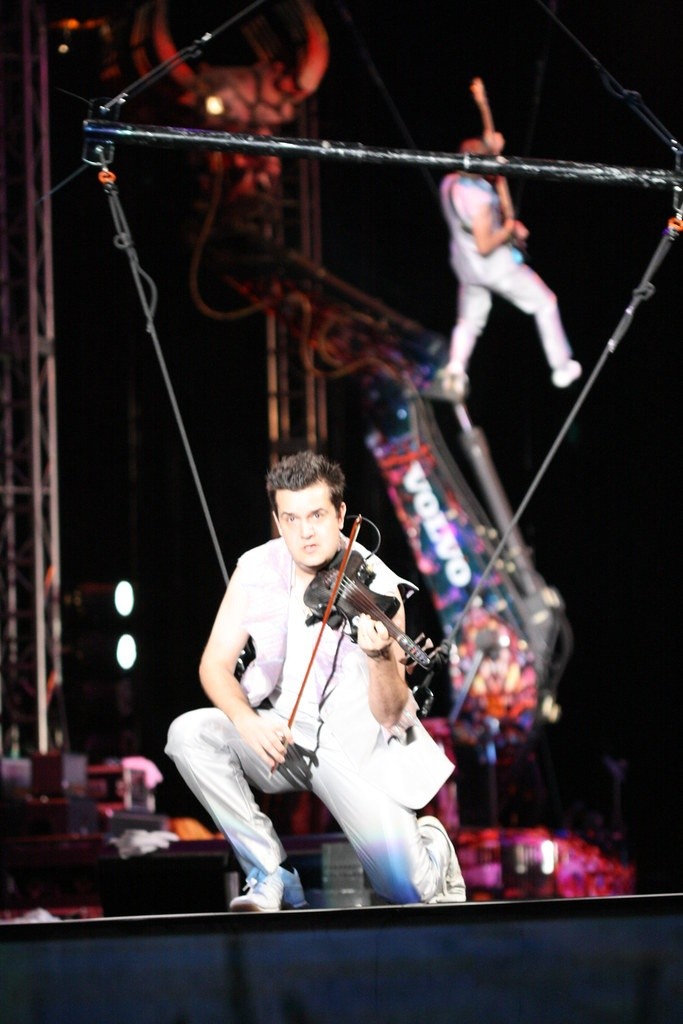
<box><xmin>470</xmin><ymin>77</ymin><xmax>531</xmax><ymax>256</ymax></box>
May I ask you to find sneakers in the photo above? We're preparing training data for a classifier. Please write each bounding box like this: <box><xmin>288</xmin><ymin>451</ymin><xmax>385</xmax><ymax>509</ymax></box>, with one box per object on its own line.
<box><xmin>229</xmin><ymin>863</ymin><xmax>309</xmax><ymax>912</ymax></box>
<box><xmin>419</xmin><ymin>814</ymin><xmax>468</xmax><ymax>906</ymax></box>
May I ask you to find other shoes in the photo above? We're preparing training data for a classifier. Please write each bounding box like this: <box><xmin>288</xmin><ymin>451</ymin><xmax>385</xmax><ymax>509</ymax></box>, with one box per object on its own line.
<box><xmin>553</xmin><ymin>359</ymin><xmax>582</xmax><ymax>388</ymax></box>
<box><xmin>442</xmin><ymin>370</ymin><xmax>468</xmax><ymax>400</ymax></box>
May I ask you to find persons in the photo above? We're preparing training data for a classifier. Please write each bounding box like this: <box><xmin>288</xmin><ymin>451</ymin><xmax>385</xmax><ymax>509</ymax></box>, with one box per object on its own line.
<box><xmin>438</xmin><ymin>137</ymin><xmax>581</xmax><ymax>395</ymax></box>
<box><xmin>162</xmin><ymin>449</ymin><xmax>467</xmax><ymax>912</ymax></box>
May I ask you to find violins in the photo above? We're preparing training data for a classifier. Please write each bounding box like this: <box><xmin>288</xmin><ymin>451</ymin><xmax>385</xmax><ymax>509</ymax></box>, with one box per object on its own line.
<box><xmin>302</xmin><ymin>547</ymin><xmax>452</xmax><ymax>676</ymax></box>
